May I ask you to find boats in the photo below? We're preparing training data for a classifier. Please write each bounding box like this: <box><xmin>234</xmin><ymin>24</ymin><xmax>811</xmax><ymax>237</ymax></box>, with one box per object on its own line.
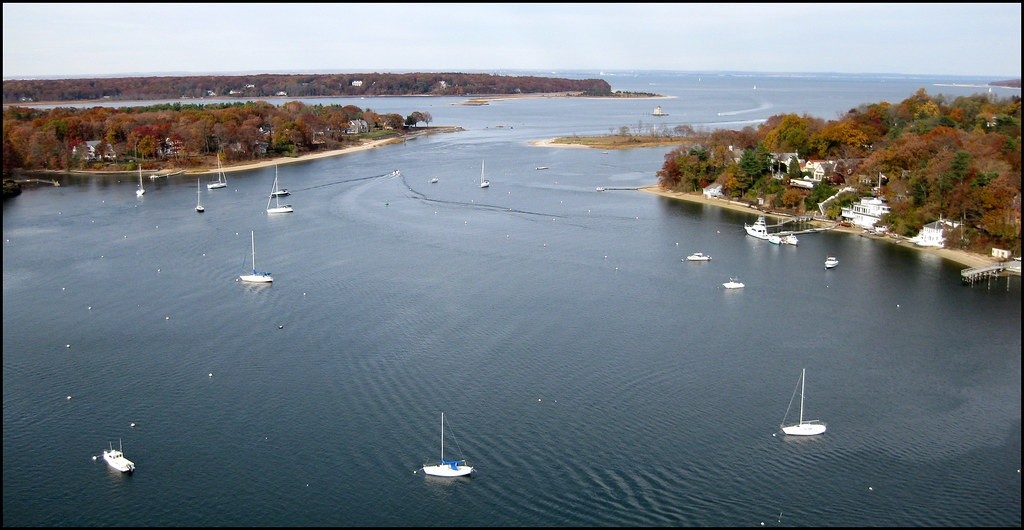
<box><xmin>104</xmin><ymin>448</ymin><xmax>136</xmax><ymax>472</ymax></box>
<box><xmin>536</xmin><ymin>167</ymin><xmax>548</xmax><ymax>170</ymax></box>
<box><xmin>687</xmin><ymin>252</ymin><xmax>710</xmax><ymax>262</ymax></box>
<box><xmin>768</xmin><ymin>235</ymin><xmax>782</xmax><ymax>245</ymax></box>
<box><xmin>431</xmin><ymin>177</ymin><xmax>439</xmax><ymax>183</ymax></box>
<box><xmin>786</xmin><ymin>234</ymin><xmax>798</xmax><ymax>245</ymax></box>
<box><xmin>597</xmin><ymin>186</ymin><xmax>604</xmax><ymax>192</ymax></box>
<box><xmin>393</xmin><ymin>169</ymin><xmax>399</xmax><ymax>175</ymax></box>
<box><xmin>824</xmin><ymin>257</ymin><xmax>839</xmax><ymax>270</ymax></box>
<box><xmin>722</xmin><ymin>276</ymin><xmax>744</xmax><ymax>290</ymax></box>
<box><xmin>744</xmin><ymin>212</ymin><xmax>770</xmax><ymax>240</ymax></box>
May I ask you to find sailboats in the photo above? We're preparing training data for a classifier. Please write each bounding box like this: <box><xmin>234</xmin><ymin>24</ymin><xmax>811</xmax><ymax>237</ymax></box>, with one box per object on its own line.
<box><xmin>266</xmin><ymin>177</ymin><xmax>292</xmax><ymax>213</ymax></box>
<box><xmin>135</xmin><ymin>164</ymin><xmax>147</xmax><ymax>195</ymax></box>
<box><xmin>778</xmin><ymin>367</ymin><xmax>828</xmax><ymax>437</ymax></box>
<box><xmin>420</xmin><ymin>411</ymin><xmax>473</xmax><ymax>478</ymax></box>
<box><xmin>237</xmin><ymin>231</ymin><xmax>273</xmax><ymax>283</ymax></box>
<box><xmin>207</xmin><ymin>154</ymin><xmax>228</xmax><ymax>190</ymax></box>
<box><xmin>195</xmin><ymin>177</ymin><xmax>205</xmax><ymax>212</ymax></box>
<box><xmin>481</xmin><ymin>159</ymin><xmax>490</xmax><ymax>187</ymax></box>
<box><xmin>269</xmin><ymin>163</ymin><xmax>290</xmax><ymax>199</ymax></box>
